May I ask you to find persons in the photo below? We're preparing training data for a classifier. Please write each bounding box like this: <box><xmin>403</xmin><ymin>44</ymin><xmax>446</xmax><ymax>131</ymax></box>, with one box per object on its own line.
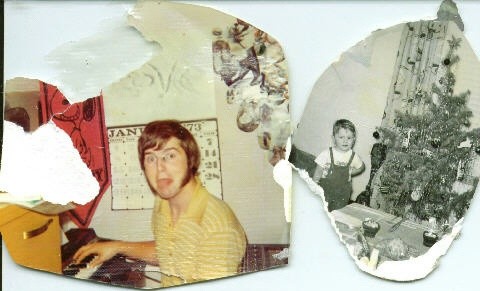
<box><xmin>311</xmin><ymin>118</ymin><xmax>365</xmax><ymax>211</ymax></box>
<box><xmin>72</xmin><ymin>118</ymin><xmax>247</xmax><ymax>288</ymax></box>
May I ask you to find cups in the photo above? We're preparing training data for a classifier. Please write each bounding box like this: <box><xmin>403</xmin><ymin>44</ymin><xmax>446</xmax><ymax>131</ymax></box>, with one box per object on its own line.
<box><xmin>362</xmin><ymin>221</ymin><xmax>380</xmax><ymax>238</ymax></box>
<box><xmin>423</xmin><ymin>231</ymin><xmax>438</xmax><ymax>247</ymax></box>
